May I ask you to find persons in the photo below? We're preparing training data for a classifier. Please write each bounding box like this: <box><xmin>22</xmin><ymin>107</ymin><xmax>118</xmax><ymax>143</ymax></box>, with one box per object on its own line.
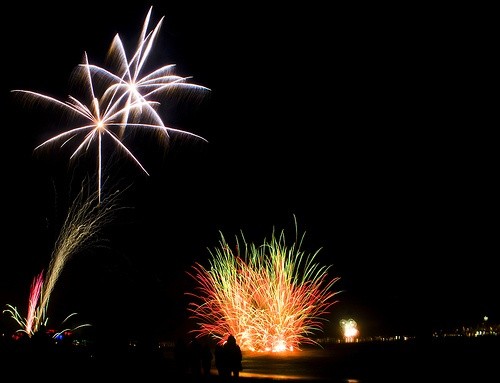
<box><xmin>169</xmin><ymin>333</ymin><xmax>243</xmax><ymax>380</ymax></box>
